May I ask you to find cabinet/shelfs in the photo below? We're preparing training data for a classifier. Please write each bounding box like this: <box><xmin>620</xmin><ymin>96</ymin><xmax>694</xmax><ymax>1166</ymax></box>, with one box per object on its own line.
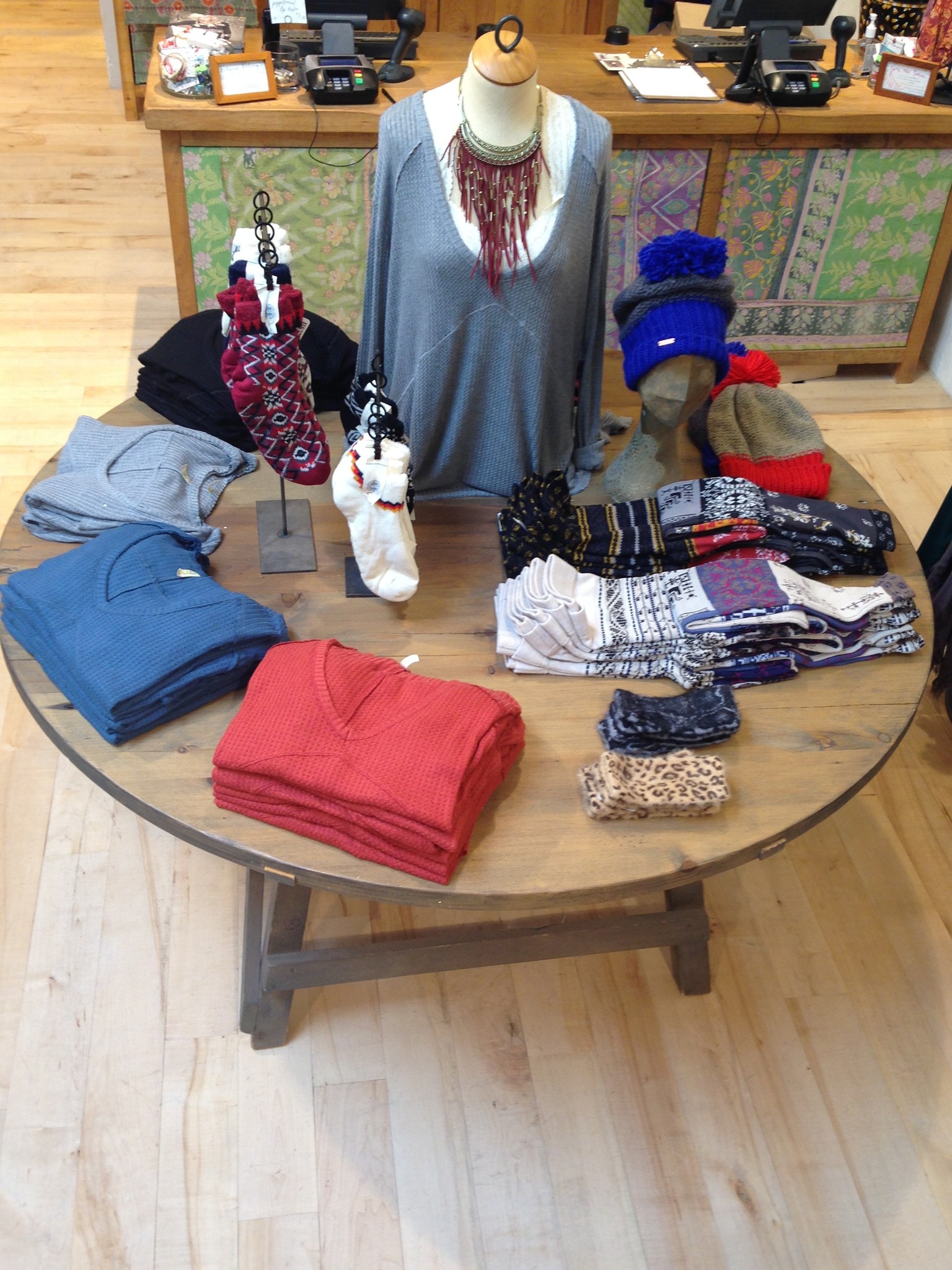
<box><xmin>142</xmin><ymin>25</ymin><xmax>951</xmax><ymax>385</ymax></box>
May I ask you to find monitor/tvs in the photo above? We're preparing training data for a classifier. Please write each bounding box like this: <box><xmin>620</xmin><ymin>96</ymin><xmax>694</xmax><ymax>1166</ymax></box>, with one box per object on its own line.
<box><xmin>703</xmin><ymin>0</ymin><xmax>836</xmax><ymax>74</ymax></box>
<box><xmin>297</xmin><ymin>0</ymin><xmax>406</xmax><ymax>91</ymax></box>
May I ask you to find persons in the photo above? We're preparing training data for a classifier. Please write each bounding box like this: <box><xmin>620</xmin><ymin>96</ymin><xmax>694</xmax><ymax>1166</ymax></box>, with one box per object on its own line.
<box><xmin>347</xmin><ymin>13</ymin><xmax>603</xmax><ymax>503</ymax></box>
<box><xmin>602</xmin><ymin>308</ymin><xmax>722</xmax><ymax>505</ymax></box>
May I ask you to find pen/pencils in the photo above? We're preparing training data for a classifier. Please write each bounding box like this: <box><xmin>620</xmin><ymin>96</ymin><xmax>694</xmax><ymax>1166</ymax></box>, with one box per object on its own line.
<box><xmin>788</xmin><ymin>39</ymin><xmax>810</xmax><ymax>43</ymax></box>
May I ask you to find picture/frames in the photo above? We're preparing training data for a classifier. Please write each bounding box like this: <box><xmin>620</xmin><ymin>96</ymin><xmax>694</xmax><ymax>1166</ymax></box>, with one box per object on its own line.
<box><xmin>872</xmin><ymin>52</ymin><xmax>941</xmax><ymax>106</ymax></box>
<box><xmin>209</xmin><ymin>50</ymin><xmax>279</xmax><ymax>106</ymax></box>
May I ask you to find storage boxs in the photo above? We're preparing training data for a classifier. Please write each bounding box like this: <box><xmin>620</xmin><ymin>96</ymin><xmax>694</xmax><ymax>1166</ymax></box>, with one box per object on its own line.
<box><xmin>155</xmin><ymin>33</ymin><xmax>234</xmax><ymax>101</ymax></box>
<box><xmin>261</xmin><ymin>39</ymin><xmax>304</xmax><ymax>96</ymax></box>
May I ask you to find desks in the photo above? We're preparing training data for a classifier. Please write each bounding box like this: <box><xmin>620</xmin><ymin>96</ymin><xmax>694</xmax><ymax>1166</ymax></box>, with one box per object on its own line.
<box><xmin>1</xmin><ymin>330</ymin><xmax>932</xmax><ymax>1052</ymax></box>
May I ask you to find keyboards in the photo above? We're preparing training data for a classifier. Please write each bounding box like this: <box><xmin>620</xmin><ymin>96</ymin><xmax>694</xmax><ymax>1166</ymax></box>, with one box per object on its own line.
<box><xmin>279</xmin><ymin>31</ymin><xmax>419</xmax><ymax>59</ymax></box>
<box><xmin>673</xmin><ymin>34</ymin><xmax>827</xmax><ymax>62</ymax></box>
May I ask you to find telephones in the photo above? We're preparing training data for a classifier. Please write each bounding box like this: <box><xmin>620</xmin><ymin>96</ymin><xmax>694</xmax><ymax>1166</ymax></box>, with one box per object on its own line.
<box><xmin>724</xmin><ymin>32</ymin><xmax>764</xmax><ymax>103</ymax></box>
